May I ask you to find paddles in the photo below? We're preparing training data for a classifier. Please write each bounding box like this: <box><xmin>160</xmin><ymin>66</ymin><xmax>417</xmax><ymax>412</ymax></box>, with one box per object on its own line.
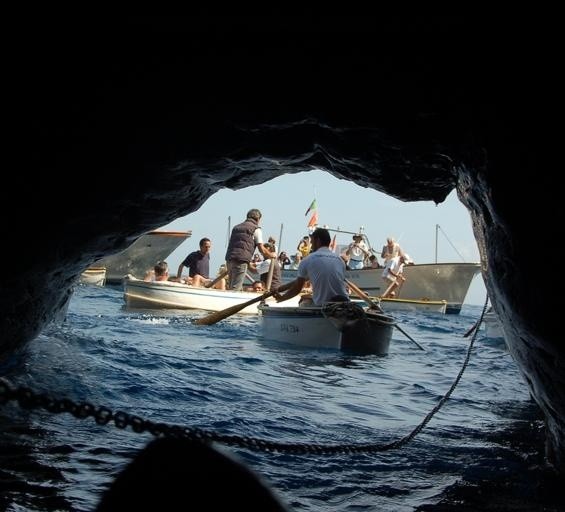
<box><xmin>205</xmin><ymin>273</ymin><xmax>229</xmax><ymax>288</ymax></box>
<box><xmin>344</xmin><ymin>279</ymin><xmax>424</xmax><ymax>350</ymax></box>
<box><xmin>191</xmin><ymin>280</ymin><xmax>300</xmax><ymax>325</ymax></box>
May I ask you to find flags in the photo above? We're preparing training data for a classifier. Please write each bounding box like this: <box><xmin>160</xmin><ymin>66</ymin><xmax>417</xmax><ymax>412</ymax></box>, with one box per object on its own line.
<box><xmin>306</xmin><ymin>212</ymin><xmax>319</xmax><ymax>228</ymax></box>
<box><xmin>304</xmin><ymin>199</ymin><xmax>318</xmax><ymax>217</ymax></box>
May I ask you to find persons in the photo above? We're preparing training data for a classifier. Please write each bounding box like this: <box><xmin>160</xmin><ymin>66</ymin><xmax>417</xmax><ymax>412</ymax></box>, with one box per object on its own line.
<box><xmin>272</xmin><ymin>226</ymin><xmax>350</xmax><ymax>308</ymax></box>
<box><xmin>224</xmin><ymin>207</ymin><xmax>278</xmax><ymax>292</ymax></box>
<box><xmin>142</xmin><ymin>233</ymin><xmax>413</xmax><ymax>300</ymax></box>
